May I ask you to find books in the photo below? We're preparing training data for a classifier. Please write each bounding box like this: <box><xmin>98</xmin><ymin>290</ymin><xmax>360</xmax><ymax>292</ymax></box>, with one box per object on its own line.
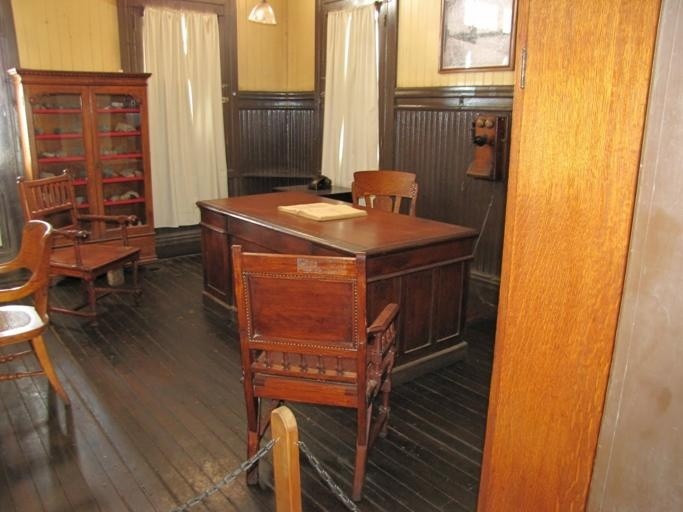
<box><xmin>277</xmin><ymin>199</ymin><xmax>368</xmax><ymax>222</ymax></box>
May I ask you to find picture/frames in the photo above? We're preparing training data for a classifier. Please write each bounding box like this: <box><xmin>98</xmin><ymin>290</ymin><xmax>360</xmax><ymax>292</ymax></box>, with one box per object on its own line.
<box><xmin>439</xmin><ymin>1</ymin><xmax>514</xmax><ymax>71</ymax></box>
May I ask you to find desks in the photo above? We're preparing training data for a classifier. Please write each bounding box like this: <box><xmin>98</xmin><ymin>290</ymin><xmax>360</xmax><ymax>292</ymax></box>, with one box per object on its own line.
<box><xmin>197</xmin><ymin>192</ymin><xmax>478</xmax><ymax>381</ymax></box>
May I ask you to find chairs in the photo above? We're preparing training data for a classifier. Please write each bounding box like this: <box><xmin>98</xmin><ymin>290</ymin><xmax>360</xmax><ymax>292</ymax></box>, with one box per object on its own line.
<box><xmin>229</xmin><ymin>244</ymin><xmax>400</xmax><ymax>499</ymax></box>
<box><xmin>1</xmin><ymin>221</ymin><xmax>71</xmax><ymax>407</ymax></box>
<box><xmin>17</xmin><ymin>176</ymin><xmax>145</xmax><ymax>332</ymax></box>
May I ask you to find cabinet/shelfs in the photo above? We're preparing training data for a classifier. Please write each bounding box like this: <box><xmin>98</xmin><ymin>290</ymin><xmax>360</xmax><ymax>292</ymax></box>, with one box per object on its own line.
<box><xmin>9</xmin><ymin>67</ymin><xmax>159</xmax><ymax>268</ymax></box>
<box><xmin>352</xmin><ymin>171</ymin><xmax>419</xmax><ymax>220</ymax></box>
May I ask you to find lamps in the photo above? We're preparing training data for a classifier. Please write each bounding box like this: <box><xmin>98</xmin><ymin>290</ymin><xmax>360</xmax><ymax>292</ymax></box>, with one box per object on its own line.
<box><xmin>247</xmin><ymin>1</ymin><xmax>278</xmax><ymax>27</ymax></box>
